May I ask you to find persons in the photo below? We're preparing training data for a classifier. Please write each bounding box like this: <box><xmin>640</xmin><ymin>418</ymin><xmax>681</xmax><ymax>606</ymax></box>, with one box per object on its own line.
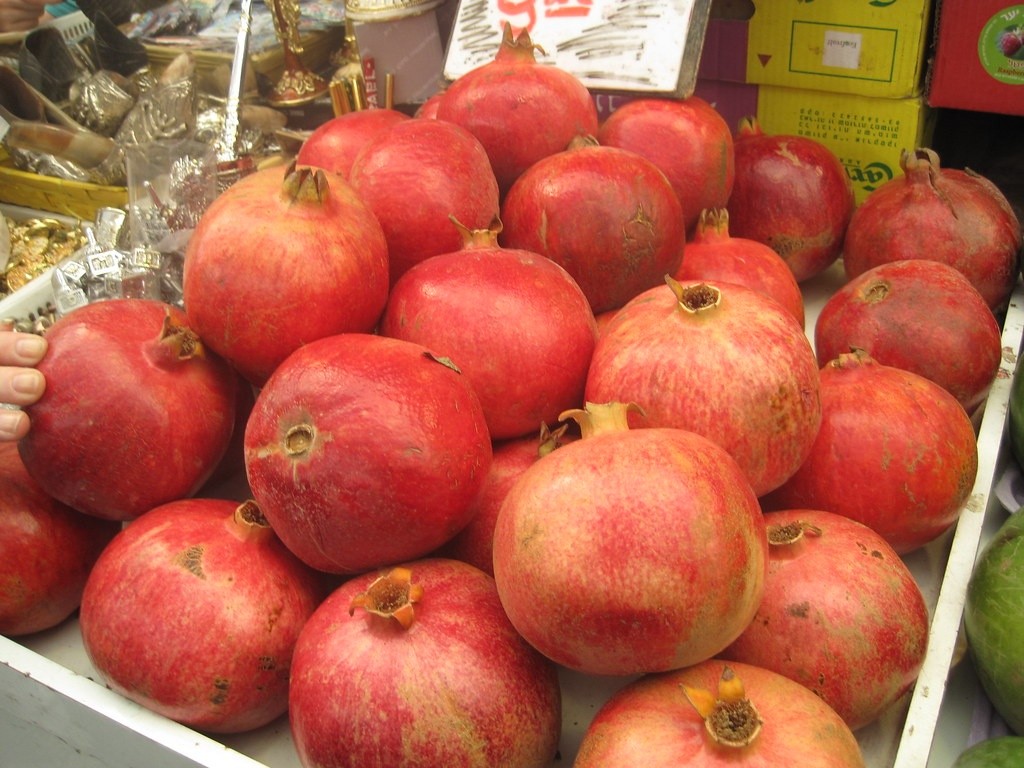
<box><xmin>0</xmin><ymin>0</ymin><xmax>79</xmax><ymax>33</ymax></box>
<box><xmin>0</xmin><ymin>320</ymin><xmax>46</xmax><ymax>440</ymax></box>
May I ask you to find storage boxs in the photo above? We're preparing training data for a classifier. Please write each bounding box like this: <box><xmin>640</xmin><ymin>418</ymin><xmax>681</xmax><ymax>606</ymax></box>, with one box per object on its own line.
<box><xmin>584</xmin><ymin>0</ymin><xmax>1024</xmax><ymax>202</ymax></box>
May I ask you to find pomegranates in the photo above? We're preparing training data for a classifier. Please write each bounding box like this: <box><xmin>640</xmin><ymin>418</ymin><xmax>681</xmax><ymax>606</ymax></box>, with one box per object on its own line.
<box><xmin>0</xmin><ymin>19</ymin><xmax>1023</xmax><ymax>768</ymax></box>
